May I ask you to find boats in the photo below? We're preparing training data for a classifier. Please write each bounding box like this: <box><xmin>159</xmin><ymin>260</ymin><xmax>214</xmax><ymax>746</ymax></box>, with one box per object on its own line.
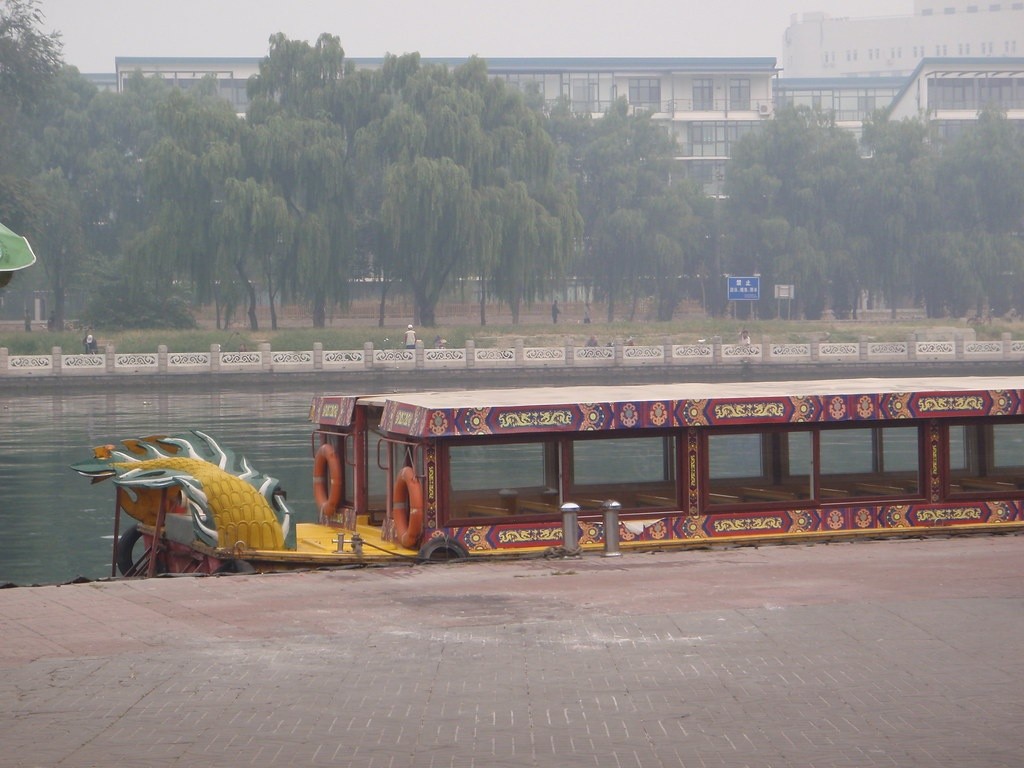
<box><xmin>70</xmin><ymin>372</ymin><xmax>1024</xmax><ymax>579</ymax></box>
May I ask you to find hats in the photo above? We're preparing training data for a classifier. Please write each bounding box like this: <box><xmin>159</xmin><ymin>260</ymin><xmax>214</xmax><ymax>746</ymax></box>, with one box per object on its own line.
<box><xmin>87</xmin><ymin>335</ymin><xmax>93</xmax><ymax>343</ymax></box>
<box><xmin>0</xmin><ymin>222</ymin><xmax>37</xmax><ymax>272</ymax></box>
<box><xmin>408</xmin><ymin>324</ymin><xmax>413</xmax><ymax>329</ymax></box>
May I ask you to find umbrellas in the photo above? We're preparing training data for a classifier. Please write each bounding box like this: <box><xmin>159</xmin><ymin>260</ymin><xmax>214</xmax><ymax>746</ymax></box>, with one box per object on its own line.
<box><xmin>0</xmin><ymin>223</ymin><xmax>36</xmax><ymax>287</ymax></box>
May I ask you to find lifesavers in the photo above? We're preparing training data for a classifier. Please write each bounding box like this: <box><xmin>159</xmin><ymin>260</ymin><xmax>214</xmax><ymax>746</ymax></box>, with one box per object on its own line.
<box><xmin>313</xmin><ymin>443</ymin><xmax>341</xmax><ymax>516</ymax></box>
<box><xmin>391</xmin><ymin>465</ymin><xmax>423</xmax><ymax>547</ymax></box>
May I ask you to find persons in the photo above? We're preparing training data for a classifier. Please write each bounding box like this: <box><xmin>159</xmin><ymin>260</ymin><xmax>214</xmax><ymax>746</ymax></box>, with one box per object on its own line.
<box><xmin>25</xmin><ymin>310</ymin><xmax>99</xmax><ymax>354</ymax></box>
<box><xmin>736</xmin><ymin>329</ymin><xmax>750</xmax><ymax>364</ymax></box>
<box><xmin>583</xmin><ymin>303</ymin><xmax>634</xmax><ymax>347</ymax></box>
<box><xmin>551</xmin><ymin>299</ymin><xmax>561</xmax><ymax>324</ymax></box>
<box><xmin>404</xmin><ymin>324</ymin><xmax>418</xmax><ymax>349</ymax></box>
